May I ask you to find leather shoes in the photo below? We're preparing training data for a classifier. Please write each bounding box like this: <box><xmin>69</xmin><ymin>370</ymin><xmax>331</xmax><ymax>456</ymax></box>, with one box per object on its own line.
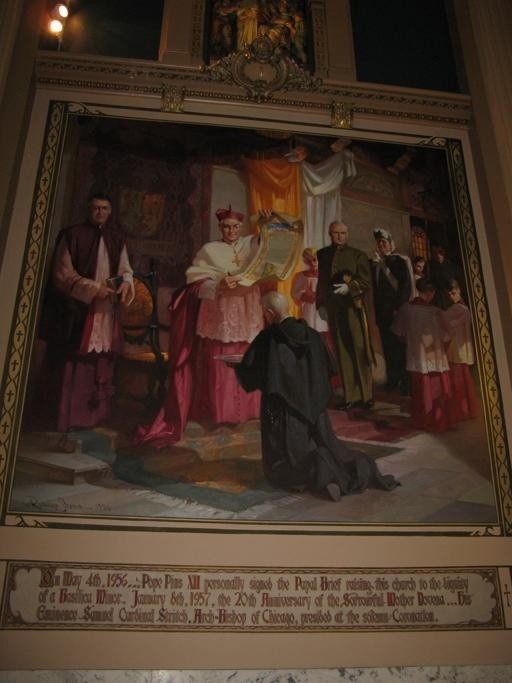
<box><xmin>335</xmin><ymin>398</ymin><xmax>375</xmax><ymax>411</ymax></box>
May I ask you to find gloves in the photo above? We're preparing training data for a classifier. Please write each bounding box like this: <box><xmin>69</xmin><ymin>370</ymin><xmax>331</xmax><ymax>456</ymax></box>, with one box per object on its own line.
<box><xmin>333</xmin><ymin>283</ymin><xmax>350</xmax><ymax>297</ymax></box>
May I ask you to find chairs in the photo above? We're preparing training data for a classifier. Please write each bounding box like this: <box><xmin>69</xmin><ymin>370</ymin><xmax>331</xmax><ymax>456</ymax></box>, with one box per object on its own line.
<box><xmin>106</xmin><ymin>269</ymin><xmax>168</xmax><ymax>404</ymax></box>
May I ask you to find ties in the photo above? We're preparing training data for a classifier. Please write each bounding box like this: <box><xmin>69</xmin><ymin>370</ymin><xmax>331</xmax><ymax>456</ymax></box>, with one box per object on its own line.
<box><xmin>331</xmin><ymin>245</ymin><xmax>341</xmax><ymax>278</ymax></box>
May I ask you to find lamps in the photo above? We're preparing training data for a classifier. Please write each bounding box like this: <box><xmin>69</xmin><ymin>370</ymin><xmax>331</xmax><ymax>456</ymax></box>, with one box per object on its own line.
<box><xmin>42</xmin><ymin>2</ymin><xmax>74</xmax><ymax>36</ymax></box>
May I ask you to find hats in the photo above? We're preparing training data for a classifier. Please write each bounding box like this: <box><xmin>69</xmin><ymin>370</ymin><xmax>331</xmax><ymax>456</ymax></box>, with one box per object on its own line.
<box><xmin>373</xmin><ymin>228</ymin><xmax>391</xmax><ymax>243</ymax></box>
<box><xmin>216</xmin><ymin>203</ymin><xmax>244</xmax><ymax>223</ymax></box>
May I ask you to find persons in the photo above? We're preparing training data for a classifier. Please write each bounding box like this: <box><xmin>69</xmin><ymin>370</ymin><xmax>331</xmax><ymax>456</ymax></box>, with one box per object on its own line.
<box><xmin>34</xmin><ymin>189</ymin><xmax>483</xmax><ymax>502</ymax></box>
<box><xmin>207</xmin><ymin>0</ymin><xmax>308</xmax><ymax>67</ymax></box>
